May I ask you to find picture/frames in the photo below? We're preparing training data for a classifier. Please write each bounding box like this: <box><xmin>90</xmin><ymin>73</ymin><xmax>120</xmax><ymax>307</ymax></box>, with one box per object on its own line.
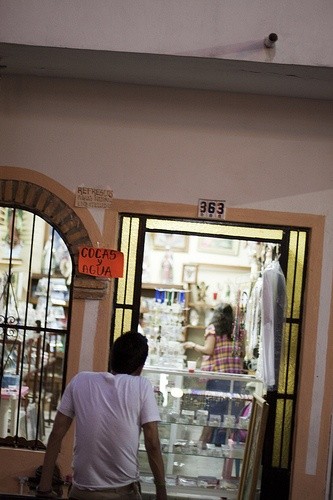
<box><xmin>152</xmin><ymin>233</ymin><xmax>191</xmax><ymax>253</ymax></box>
<box><xmin>44</xmin><ymin>221</ymin><xmax>69</xmax><ymax>271</ymax></box>
<box><xmin>181</xmin><ymin>264</ymin><xmax>198</xmax><ymax>284</ymax></box>
<box><xmin>196</xmin><ymin>236</ymin><xmax>241</xmax><ymax>256</ymax></box>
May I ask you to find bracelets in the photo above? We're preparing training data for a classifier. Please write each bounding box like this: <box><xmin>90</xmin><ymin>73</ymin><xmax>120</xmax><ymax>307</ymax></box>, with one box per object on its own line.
<box><xmin>192</xmin><ymin>343</ymin><xmax>197</xmax><ymax>351</ymax></box>
<box><xmin>36</xmin><ymin>484</ymin><xmax>53</xmax><ymax>495</ymax></box>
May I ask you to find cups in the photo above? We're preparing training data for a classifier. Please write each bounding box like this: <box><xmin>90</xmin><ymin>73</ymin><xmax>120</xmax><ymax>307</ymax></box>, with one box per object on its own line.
<box><xmin>187</xmin><ymin>361</ymin><xmax>198</xmax><ymax>372</ymax></box>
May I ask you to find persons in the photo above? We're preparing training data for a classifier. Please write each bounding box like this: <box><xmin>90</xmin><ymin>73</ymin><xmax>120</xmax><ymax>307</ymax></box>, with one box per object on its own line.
<box><xmin>36</xmin><ymin>331</ymin><xmax>168</xmax><ymax>500</ymax></box>
<box><xmin>186</xmin><ymin>303</ymin><xmax>247</xmax><ymax>449</ymax></box>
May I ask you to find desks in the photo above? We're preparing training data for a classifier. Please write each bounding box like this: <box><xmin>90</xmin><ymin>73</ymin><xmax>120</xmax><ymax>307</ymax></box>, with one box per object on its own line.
<box><xmin>0</xmin><ymin>386</ymin><xmax>30</xmax><ymax>438</ymax></box>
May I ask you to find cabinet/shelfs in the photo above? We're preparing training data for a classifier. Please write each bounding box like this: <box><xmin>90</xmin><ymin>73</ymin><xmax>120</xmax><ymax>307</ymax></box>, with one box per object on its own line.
<box><xmin>185</xmin><ymin>262</ymin><xmax>251</xmax><ymax>342</ymax></box>
<box><xmin>26</xmin><ymin>272</ymin><xmax>70</xmax><ymax>408</ymax></box>
<box><xmin>139</xmin><ymin>366</ymin><xmax>258</xmax><ymax>500</ymax></box>
